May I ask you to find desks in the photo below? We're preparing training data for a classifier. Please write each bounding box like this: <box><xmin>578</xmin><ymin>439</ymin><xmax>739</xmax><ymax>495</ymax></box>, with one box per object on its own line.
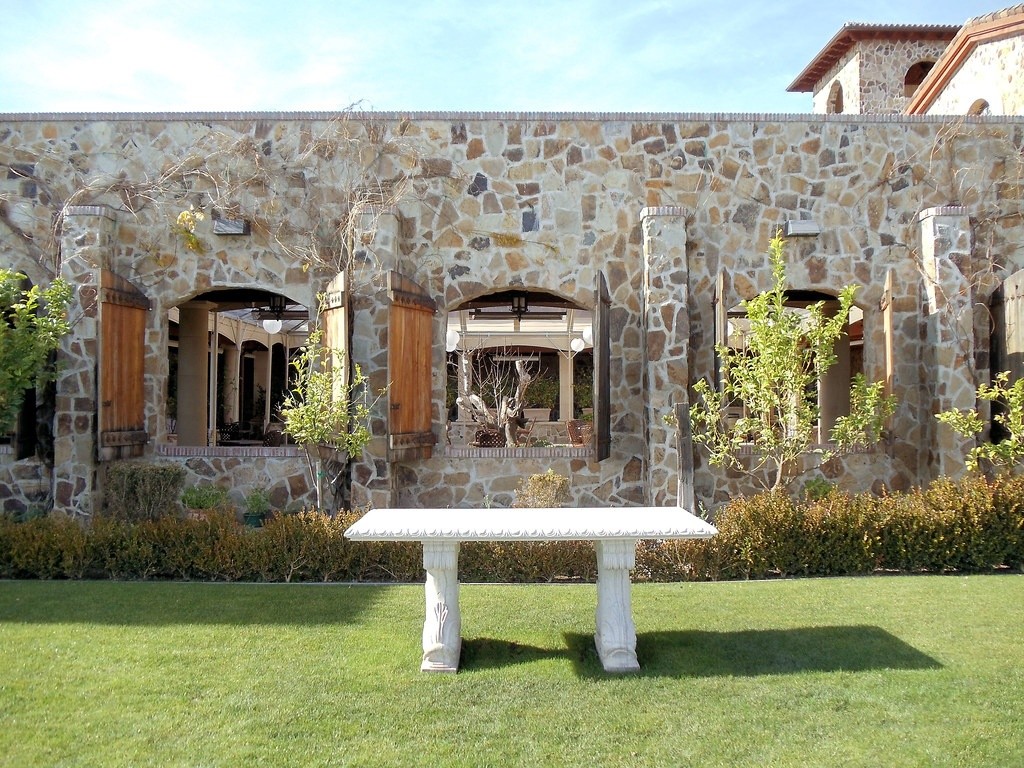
<box><xmin>344</xmin><ymin>507</ymin><xmax>719</xmax><ymax>671</ymax></box>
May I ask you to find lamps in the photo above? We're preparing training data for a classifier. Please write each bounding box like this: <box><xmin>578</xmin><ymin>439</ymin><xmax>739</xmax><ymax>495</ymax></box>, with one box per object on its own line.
<box><xmin>213</xmin><ymin>219</ymin><xmax>250</xmax><ymax>236</ymax></box>
<box><xmin>785</xmin><ymin>220</ymin><xmax>821</xmax><ymax>238</ymax></box>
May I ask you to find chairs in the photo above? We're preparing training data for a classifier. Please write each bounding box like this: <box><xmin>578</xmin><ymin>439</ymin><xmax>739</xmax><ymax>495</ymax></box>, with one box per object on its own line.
<box><xmin>218</xmin><ymin>423</ymin><xmax>283</xmax><ymax>447</ymax></box>
<box><xmin>468</xmin><ymin>418</ymin><xmax>592</xmax><ymax>448</ymax></box>
